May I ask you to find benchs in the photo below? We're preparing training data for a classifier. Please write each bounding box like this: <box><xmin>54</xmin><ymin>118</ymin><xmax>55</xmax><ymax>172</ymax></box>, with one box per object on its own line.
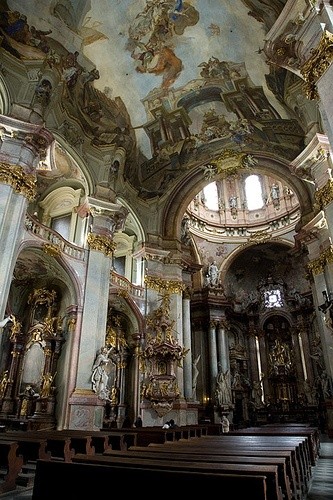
<box><xmin>1</xmin><ymin>421</ymin><xmax>321</xmax><ymax>500</ymax></box>
